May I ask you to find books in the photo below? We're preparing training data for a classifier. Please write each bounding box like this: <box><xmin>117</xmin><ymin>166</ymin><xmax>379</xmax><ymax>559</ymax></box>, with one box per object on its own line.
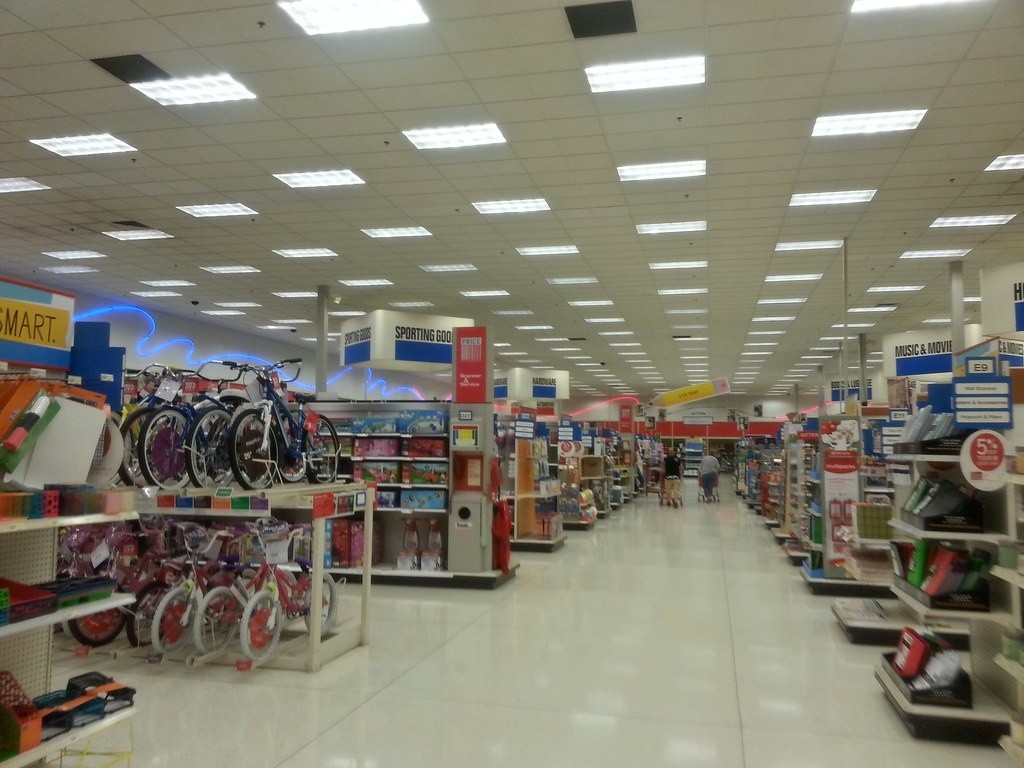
<box><xmin>903</xmin><ymin>476</ymin><xmax>987</xmax><ymax>516</ymax></box>
<box><xmin>890</xmin><ymin>540</ymin><xmax>995</xmax><ymax>595</ymax></box>
<box><xmin>899</xmin><ymin>405</ymin><xmax>964</xmax><ymax>443</ymax></box>
<box><xmin>892</xmin><ymin>628</ymin><xmax>963</xmax><ymax>691</ymax></box>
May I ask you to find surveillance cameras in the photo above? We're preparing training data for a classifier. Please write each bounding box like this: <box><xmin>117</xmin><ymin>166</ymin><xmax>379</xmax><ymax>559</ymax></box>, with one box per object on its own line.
<box><xmin>600</xmin><ymin>362</ymin><xmax>605</xmax><ymax>365</ymax></box>
<box><xmin>291</xmin><ymin>329</ymin><xmax>296</xmax><ymax>332</ymax></box>
<box><xmin>191</xmin><ymin>300</ymin><xmax>199</xmax><ymax>305</ymax></box>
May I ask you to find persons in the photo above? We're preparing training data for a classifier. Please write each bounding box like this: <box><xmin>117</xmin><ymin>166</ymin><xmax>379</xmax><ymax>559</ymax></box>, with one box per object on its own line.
<box><xmin>700</xmin><ymin>447</ymin><xmax>721</xmax><ymax>503</ymax></box>
<box><xmin>663</xmin><ymin>447</ymin><xmax>684</xmax><ymax>509</ymax></box>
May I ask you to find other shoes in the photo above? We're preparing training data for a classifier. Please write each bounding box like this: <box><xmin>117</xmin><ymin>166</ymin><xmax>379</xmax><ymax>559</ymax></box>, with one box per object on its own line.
<box><xmin>674</xmin><ymin>503</ymin><xmax>678</xmax><ymax>508</ymax></box>
<box><xmin>705</xmin><ymin>498</ymin><xmax>712</xmax><ymax>503</ymax></box>
<box><xmin>667</xmin><ymin>503</ymin><xmax>671</xmax><ymax>506</ymax></box>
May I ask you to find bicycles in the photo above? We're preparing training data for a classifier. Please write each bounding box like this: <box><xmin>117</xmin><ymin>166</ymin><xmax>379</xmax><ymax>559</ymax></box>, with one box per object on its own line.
<box><xmin>116</xmin><ymin>355</ymin><xmax>340</xmax><ymax>492</ymax></box>
<box><xmin>60</xmin><ymin>524</ymin><xmax>339</xmax><ymax>661</ymax></box>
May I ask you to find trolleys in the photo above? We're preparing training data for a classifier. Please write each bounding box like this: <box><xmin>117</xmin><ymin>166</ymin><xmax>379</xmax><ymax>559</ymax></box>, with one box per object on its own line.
<box><xmin>659</xmin><ymin>466</ymin><xmax>683</xmax><ymax>507</ymax></box>
<box><xmin>645</xmin><ymin>467</ymin><xmax>661</xmax><ymax>495</ymax></box>
<box><xmin>698</xmin><ymin>470</ymin><xmax>720</xmax><ymax>503</ymax></box>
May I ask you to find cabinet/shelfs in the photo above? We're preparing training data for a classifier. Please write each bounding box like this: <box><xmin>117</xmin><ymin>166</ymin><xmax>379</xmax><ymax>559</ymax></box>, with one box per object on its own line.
<box><xmin>510</xmin><ymin>399</ymin><xmax>708</xmax><ymax>555</ymax></box>
<box><xmin>734</xmin><ymin>363</ymin><xmax>1024</xmax><ymax>747</ymax></box>
<box><xmin>0</xmin><ymin>275</ymin><xmax>515</xmax><ymax>768</ymax></box>
